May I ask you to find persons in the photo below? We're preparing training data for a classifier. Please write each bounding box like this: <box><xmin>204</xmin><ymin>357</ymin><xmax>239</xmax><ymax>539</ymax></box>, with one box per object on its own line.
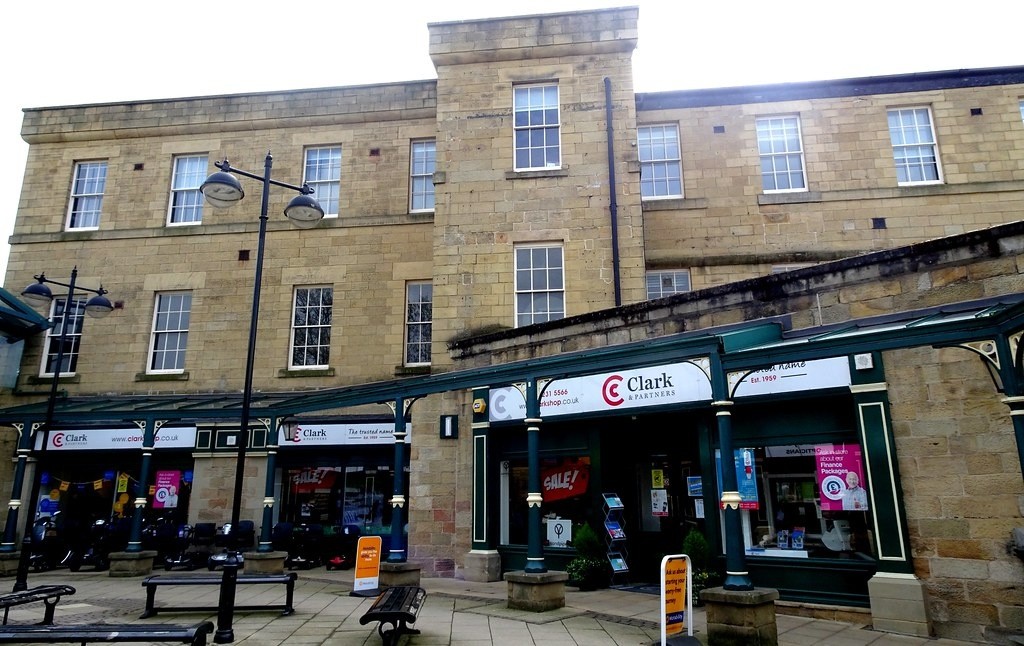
<box><xmin>842</xmin><ymin>472</ymin><xmax>868</xmax><ymax>511</ymax></box>
<box><xmin>164</xmin><ymin>486</ymin><xmax>177</xmax><ymax>507</ymax></box>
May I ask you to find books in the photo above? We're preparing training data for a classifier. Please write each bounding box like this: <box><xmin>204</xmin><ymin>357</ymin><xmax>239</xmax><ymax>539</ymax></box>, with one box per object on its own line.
<box><xmin>611</xmin><ymin>559</ymin><xmax>628</xmax><ymax>571</ymax></box>
<box><xmin>607</xmin><ymin>497</ymin><xmax>624</xmax><ymax>508</ymax></box>
<box><xmin>605</xmin><ymin>521</ymin><xmax>625</xmax><ymax>540</ymax></box>
<box><xmin>777</xmin><ymin>527</ymin><xmax>805</xmax><ymax>549</ymax></box>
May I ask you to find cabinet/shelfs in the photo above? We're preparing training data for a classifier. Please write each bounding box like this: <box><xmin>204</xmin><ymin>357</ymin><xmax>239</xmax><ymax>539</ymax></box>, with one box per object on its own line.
<box><xmin>602</xmin><ymin>493</ymin><xmax>630</xmax><ymax>588</ymax></box>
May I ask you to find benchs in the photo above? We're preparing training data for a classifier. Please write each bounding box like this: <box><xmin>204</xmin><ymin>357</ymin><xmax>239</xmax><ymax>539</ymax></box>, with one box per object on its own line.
<box><xmin>0</xmin><ymin>585</ymin><xmax>76</xmax><ymax>625</ymax></box>
<box><xmin>359</xmin><ymin>586</ymin><xmax>428</xmax><ymax>646</ymax></box>
<box><xmin>141</xmin><ymin>572</ymin><xmax>298</xmax><ymax>620</ymax></box>
<box><xmin>0</xmin><ymin>621</ymin><xmax>215</xmax><ymax>646</ymax></box>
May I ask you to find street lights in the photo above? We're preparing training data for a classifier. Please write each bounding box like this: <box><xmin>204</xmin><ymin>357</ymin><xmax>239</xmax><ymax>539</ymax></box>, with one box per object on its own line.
<box><xmin>12</xmin><ymin>265</ymin><xmax>116</xmax><ymax>593</ymax></box>
<box><xmin>198</xmin><ymin>148</ymin><xmax>324</xmax><ymax>645</ymax></box>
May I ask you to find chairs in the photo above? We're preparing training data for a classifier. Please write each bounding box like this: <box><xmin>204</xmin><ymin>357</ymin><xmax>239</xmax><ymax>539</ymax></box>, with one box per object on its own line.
<box><xmin>191</xmin><ymin>523</ymin><xmax>217</xmax><ymax>547</ymax></box>
<box><xmin>237</xmin><ymin>521</ymin><xmax>255</xmax><ymax>548</ymax></box>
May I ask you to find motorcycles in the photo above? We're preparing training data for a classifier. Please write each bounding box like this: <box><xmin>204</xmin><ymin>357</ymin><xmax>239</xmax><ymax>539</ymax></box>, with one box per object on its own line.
<box><xmin>144</xmin><ymin>510</ymin><xmax>359</xmax><ymax>570</ymax></box>
<box><xmin>29</xmin><ymin>510</ymin><xmax>63</xmax><ymax>573</ymax></box>
<box><xmin>82</xmin><ymin>510</ymin><xmax>120</xmax><ymax>570</ymax></box>
